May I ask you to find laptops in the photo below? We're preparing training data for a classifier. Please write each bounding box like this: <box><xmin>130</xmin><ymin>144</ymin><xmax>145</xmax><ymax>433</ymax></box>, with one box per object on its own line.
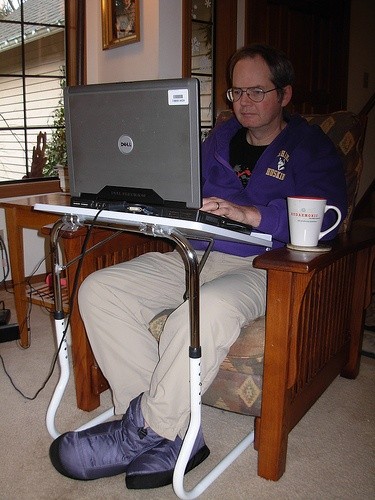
<box><xmin>63</xmin><ymin>77</ymin><xmax>253</xmax><ymax>237</ymax></box>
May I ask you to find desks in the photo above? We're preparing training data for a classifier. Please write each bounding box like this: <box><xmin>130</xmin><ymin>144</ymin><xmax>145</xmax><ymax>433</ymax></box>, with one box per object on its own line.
<box><xmin>0</xmin><ymin>191</ymin><xmax>72</xmax><ymax>348</ymax></box>
<box><xmin>34</xmin><ymin>203</ymin><xmax>274</xmax><ymax>500</ymax></box>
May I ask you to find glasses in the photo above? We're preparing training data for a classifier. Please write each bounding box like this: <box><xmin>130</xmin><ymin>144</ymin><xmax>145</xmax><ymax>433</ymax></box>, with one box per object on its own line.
<box><xmin>226</xmin><ymin>83</ymin><xmax>283</xmax><ymax>104</ymax></box>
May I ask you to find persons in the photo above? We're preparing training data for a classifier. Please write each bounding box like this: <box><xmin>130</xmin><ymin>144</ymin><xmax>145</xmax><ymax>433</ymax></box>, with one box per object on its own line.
<box><xmin>50</xmin><ymin>42</ymin><xmax>348</xmax><ymax>490</ymax></box>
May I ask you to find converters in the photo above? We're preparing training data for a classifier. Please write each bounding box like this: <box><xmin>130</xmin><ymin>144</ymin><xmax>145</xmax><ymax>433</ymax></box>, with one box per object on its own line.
<box><xmin>0</xmin><ymin>323</ymin><xmax>20</xmax><ymax>343</ymax></box>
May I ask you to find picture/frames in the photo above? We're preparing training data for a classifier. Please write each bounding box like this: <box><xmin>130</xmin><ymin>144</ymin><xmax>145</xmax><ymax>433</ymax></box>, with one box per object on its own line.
<box><xmin>101</xmin><ymin>0</ymin><xmax>140</xmax><ymax>51</ymax></box>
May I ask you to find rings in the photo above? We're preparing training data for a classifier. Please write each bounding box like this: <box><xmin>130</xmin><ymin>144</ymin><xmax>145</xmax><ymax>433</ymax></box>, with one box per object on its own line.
<box><xmin>215</xmin><ymin>201</ymin><xmax>220</xmax><ymax>210</ymax></box>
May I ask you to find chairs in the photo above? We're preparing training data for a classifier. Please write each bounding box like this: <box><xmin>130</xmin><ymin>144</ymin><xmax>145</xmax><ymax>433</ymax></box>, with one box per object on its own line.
<box><xmin>40</xmin><ymin>112</ymin><xmax>375</xmax><ymax>482</ymax></box>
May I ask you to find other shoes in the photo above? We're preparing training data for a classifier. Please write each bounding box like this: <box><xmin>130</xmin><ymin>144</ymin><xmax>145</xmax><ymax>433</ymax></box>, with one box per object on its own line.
<box><xmin>125</xmin><ymin>424</ymin><xmax>211</xmax><ymax>489</ymax></box>
<box><xmin>48</xmin><ymin>393</ymin><xmax>164</xmax><ymax>481</ymax></box>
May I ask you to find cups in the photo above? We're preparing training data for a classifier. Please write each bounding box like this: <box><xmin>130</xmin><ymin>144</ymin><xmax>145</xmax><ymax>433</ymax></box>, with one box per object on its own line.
<box><xmin>287</xmin><ymin>196</ymin><xmax>341</xmax><ymax>247</ymax></box>
